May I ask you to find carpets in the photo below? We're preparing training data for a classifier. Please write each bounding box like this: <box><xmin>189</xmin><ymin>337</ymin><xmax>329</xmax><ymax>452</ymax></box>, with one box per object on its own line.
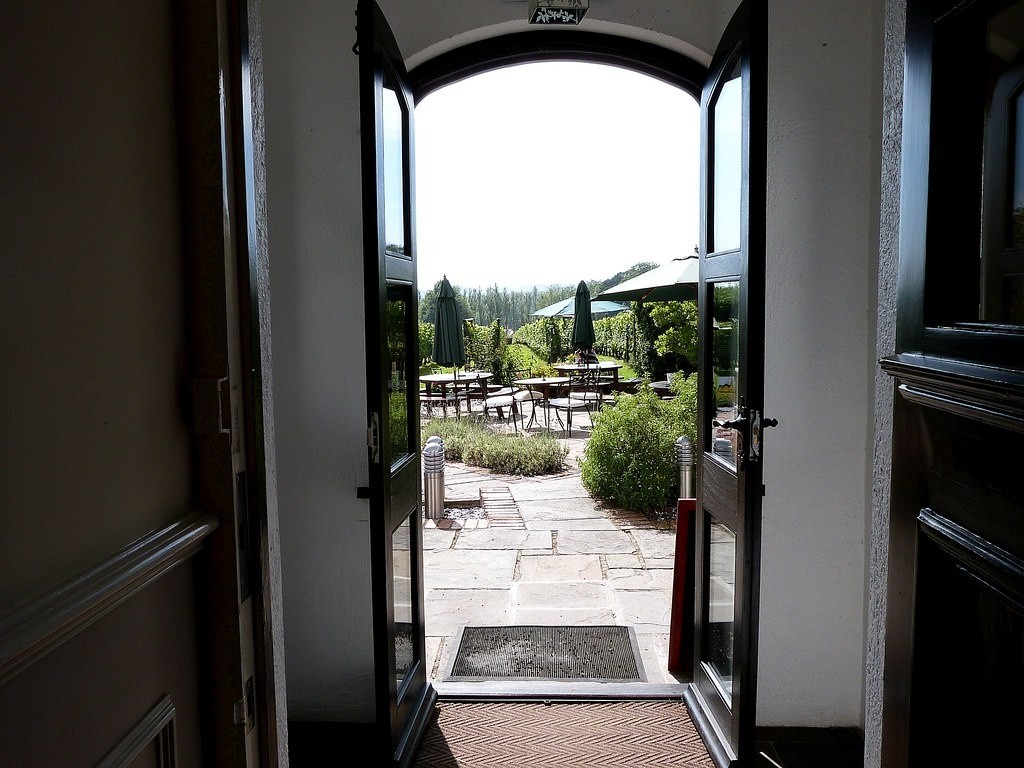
<box><xmin>405</xmin><ymin>701</ymin><xmax>716</xmax><ymax>768</ymax></box>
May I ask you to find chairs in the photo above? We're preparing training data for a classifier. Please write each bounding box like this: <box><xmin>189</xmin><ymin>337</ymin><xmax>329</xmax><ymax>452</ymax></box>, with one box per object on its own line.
<box><xmin>420</xmin><ymin>361</ymin><xmax>625</xmax><ymax>437</ymax></box>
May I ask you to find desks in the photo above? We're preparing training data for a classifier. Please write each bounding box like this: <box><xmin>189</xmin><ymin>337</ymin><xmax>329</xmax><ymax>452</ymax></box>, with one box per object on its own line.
<box><xmin>553</xmin><ymin>362</ymin><xmax>623</xmax><ymax>395</ymax></box>
<box><xmin>513</xmin><ymin>377</ymin><xmax>572</xmax><ymax>431</ymax></box>
<box><xmin>420</xmin><ymin>373</ymin><xmax>493</xmax><ymax>417</ymax></box>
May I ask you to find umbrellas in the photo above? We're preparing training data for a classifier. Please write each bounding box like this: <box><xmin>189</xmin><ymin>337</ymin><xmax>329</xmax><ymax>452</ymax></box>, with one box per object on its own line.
<box><xmin>530</xmin><ymin>296</ymin><xmax>631</xmax><ymax>318</ymax></box>
<box><xmin>570</xmin><ymin>280</ymin><xmax>596</xmax><ymax>371</ymax></box>
<box><xmin>588</xmin><ymin>244</ymin><xmax>699</xmax><ymax>301</ymax></box>
<box><xmin>433</xmin><ymin>274</ymin><xmax>466</xmax><ymax>387</ymax></box>
